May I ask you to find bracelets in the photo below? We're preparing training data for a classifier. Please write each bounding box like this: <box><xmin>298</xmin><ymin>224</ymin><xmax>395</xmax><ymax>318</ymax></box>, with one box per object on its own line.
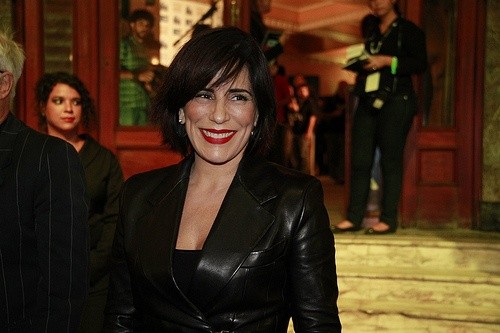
<box><xmin>391</xmin><ymin>56</ymin><xmax>398</xmax><ymax>74</ymax></box>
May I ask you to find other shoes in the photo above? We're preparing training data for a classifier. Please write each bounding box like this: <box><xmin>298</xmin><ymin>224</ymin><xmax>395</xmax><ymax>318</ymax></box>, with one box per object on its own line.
<box><xmin>332</xmin><ymin>220</ymin><xmax>361</xmax><ymax>232</ymax></box>
<box><xmin>366</xmin><ymin>221</ymin><xmax>396</xmax><ymax>234</ymax></box>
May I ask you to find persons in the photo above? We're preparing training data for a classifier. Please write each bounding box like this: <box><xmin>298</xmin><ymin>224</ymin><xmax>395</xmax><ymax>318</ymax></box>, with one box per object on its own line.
<box><xmin>102</xmin><ymin>27</ymin><xmax>341</xmax><ymax>333</ymax></box>
<box><xmin>35</xmin><ymin>71</ymin><xmax>123</xmax><ymax>333</ymax></box>
<box><xmin>329</xmin><ymin>0</ymin><xmax>427</xmax><ymax>235</ymax></box>
<box><xmin>118</xmin><ymin>9</ymin><xmax>156</xmax><ymax>127</ymax></box>
<box><xmin>0</xmin><ymin>30</ymin><xmax>91</xmax><ymax>333</ymax></box>
<box><xmin>268</xmin><ymin>63</ymin><xmax>348</xmax><ymax>178</ymax></box>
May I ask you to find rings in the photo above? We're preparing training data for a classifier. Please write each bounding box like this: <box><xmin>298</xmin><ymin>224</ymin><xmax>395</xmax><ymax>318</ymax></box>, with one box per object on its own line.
<box><xmin>372</xmin><ymin>66</ymin><xmax>377</xmax><ymax>71</ymax></box>
